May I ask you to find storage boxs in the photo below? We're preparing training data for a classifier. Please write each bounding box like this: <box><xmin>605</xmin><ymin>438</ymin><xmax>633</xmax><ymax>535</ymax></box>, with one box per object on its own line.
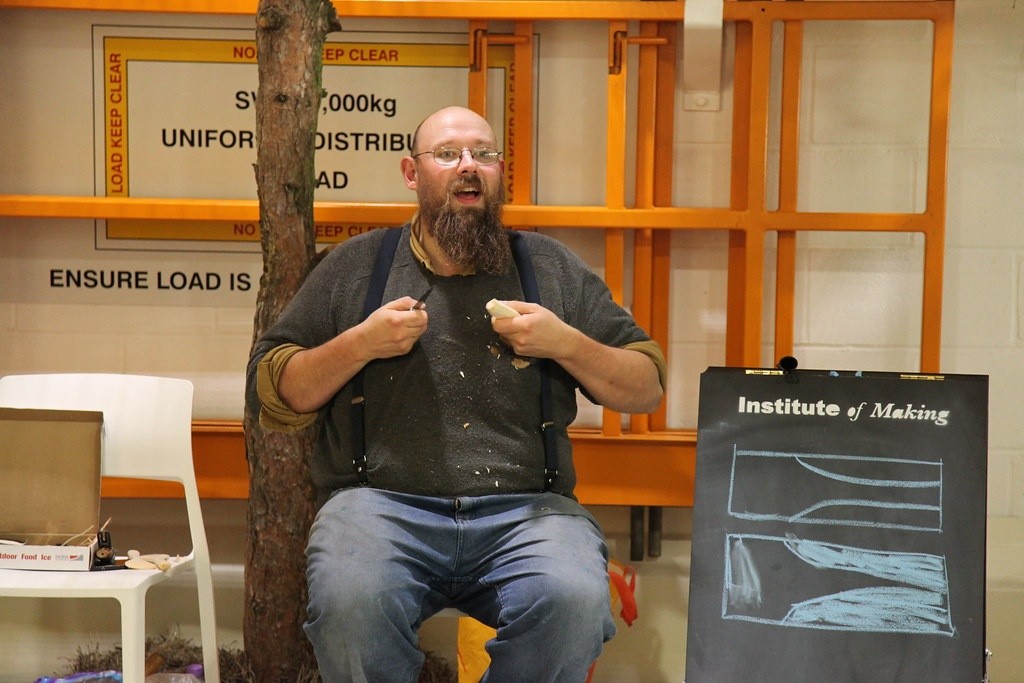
<box><xmin>0</xmin><ymin>407</ymin><xmax>105</xmax><ymax>578</ymax></box>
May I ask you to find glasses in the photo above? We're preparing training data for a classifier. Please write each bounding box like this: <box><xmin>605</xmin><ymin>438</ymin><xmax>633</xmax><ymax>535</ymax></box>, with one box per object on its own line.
<box><xmin>413</xmin><ymin>146</ymin><xmax>502</xmax><ymax>166</ymax></box>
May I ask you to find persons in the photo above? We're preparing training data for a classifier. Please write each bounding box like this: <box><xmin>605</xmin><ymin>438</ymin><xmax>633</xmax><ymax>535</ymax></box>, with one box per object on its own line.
<box><xmin>245</xmin><ymin>106</ymin><xmax>669</xmax><ymax>683</ymax></box>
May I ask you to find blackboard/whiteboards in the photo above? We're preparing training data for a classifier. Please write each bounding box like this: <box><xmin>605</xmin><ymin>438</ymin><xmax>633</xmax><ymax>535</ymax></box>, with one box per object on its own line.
<box><xmin>685</xmin><ymin>367</ymin><xmax>986</xmax><ymax>683</ymax></box>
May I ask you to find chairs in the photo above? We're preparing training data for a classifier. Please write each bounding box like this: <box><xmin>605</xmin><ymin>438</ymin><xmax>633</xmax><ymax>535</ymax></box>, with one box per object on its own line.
<box><xmin>0</xmin><ymin>373</ymin><xmax>223</xmax><ymax>683</ymax></box>
